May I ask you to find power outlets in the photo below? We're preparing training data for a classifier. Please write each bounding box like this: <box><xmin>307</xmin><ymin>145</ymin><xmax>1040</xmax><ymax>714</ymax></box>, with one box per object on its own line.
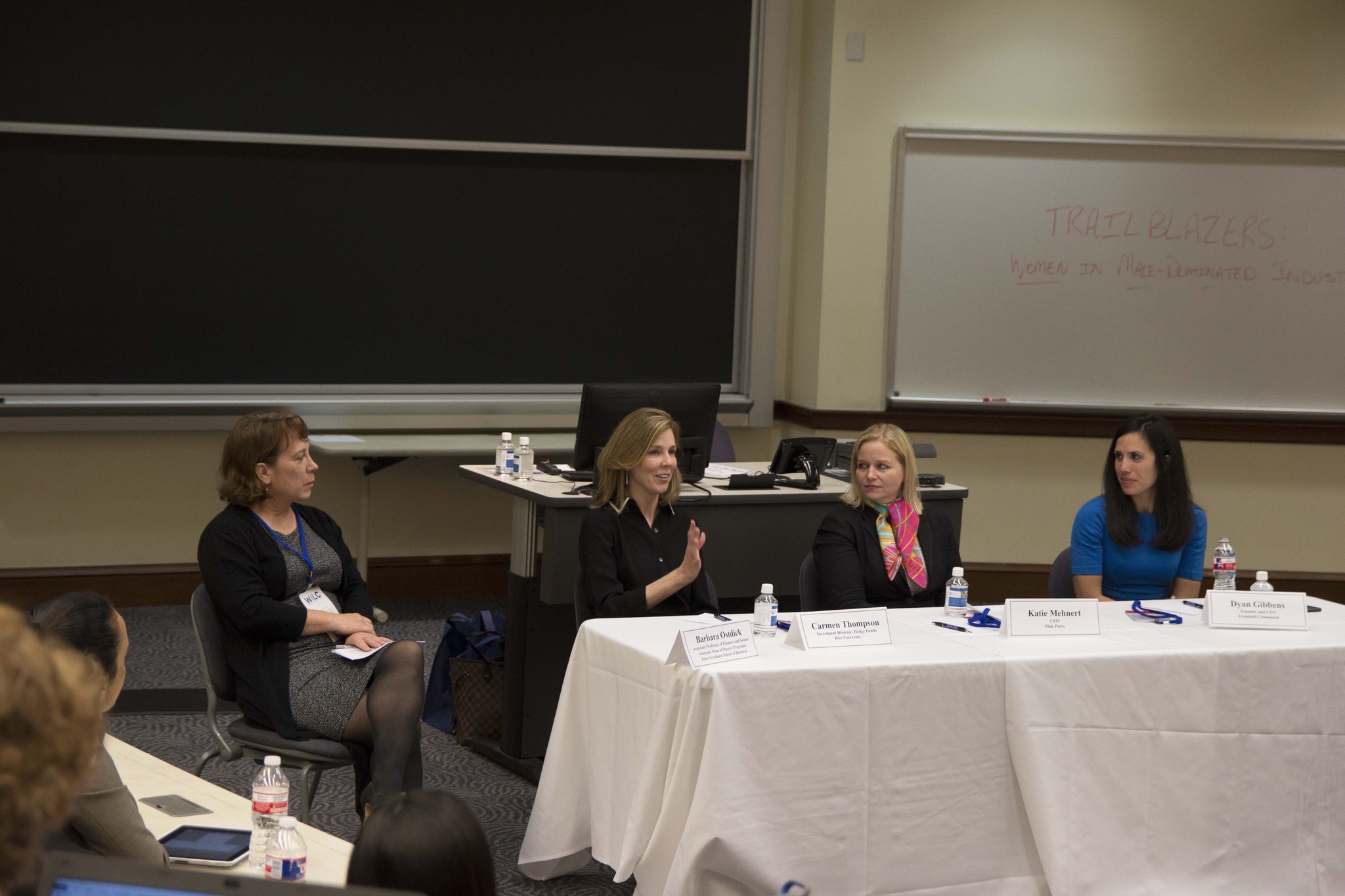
<box><xmin>845</xmin><ymin>31</ymin><xmax>866</xmax><ymax>63</ymax></box>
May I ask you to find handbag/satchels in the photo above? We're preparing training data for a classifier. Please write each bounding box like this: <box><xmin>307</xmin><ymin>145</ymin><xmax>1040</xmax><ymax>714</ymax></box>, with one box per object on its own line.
<box><xmin>420</xmin><ymin>607</ymin><xmax>501</xmax><ymax>735</ymax></box>
<box><xmin>448</xmin><ymin>629</ymin><xmax>504</xmax><ymax>746</ymax></box>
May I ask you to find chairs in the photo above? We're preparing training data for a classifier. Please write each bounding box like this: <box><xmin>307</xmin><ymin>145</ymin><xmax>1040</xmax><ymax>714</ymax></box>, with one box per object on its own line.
<box><xmin>190</xmin><ymin>579</ymin><xmax>359</xmax><ymax>829</ymax></box>
<box><xmin>711</xmin><ymin>419</ymin><xmax>739</xmax><ymax>461</ymax></box>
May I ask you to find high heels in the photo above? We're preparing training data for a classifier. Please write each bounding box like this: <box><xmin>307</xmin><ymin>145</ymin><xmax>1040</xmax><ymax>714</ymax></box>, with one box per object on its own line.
<box><xmin>360</xmin><ymin>781</ymin><xmax>382</xmax><ymax>828</ymax></box>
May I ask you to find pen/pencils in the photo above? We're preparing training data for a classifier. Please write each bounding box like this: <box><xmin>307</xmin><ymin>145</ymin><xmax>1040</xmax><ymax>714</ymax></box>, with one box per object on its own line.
<box><xmin>714</xmin><ymin>613</ymin><xmax>732</xmax><ymax>622</ymax></box>
<box><xmin>1182</xmin><ymin>600</ymin><xmax>1203</xmax><ymax>610</ymax></box>
<box><xmin>932</xmin><ymin>622</ymin><xmax>970</xmax><ymax>633</ymax></box>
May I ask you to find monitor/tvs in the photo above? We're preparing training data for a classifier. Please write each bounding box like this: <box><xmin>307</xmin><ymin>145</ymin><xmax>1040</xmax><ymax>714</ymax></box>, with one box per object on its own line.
<box><xmin>569</xmin><ymin>384</ymin><xmax>721</xmax><ymax>477</ymax></box>
<box><xmin>771</xmin><ymin>438</ymin><xmax>836</xmax><ymax>473</ymax></box>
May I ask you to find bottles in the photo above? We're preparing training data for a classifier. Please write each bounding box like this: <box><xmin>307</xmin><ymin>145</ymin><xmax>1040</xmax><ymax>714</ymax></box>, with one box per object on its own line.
<box><xmin>1250</xmin><ymin>570</ymin><xmax>1274</xmax><ymax>592</ymax></box>
<box><xmin>265</xmin><ymin>816</ymin><xmax>308</xmax><ymax>884</ymax></box>
<box><xmin>495</xmin><ymin>432</ymin><xmax>516</xmax><ymax>475</ymax></box>
<box><xmin>944</xmin><ymin>567</ymin><xmax>970</xmax><ymax>617</ymax></box>
<box><xmin>754</xmin><ymin>584</ymin><xmax>779</xmax><ymax>637</ymax></box>
<box><xmin>513</xmin><ymin>436</ymin><xmax>536</xmax><ymax>481</ymax></box>
<box><xmin>1213</xmin><ymin>537</ymin><xmax>1235</xmax><ymax>591</ymax></box>
<box><xmin>249</xmin><ymin>756</ymin><xmax>290</xmax><ymax>872</ymax></box>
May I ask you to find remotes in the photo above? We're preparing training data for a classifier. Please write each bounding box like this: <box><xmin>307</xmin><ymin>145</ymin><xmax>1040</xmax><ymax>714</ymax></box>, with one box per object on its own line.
<box><xmin>537</xmin><ymin>463</ymin><xmax>561</xmax><ymax>475</ymax></box>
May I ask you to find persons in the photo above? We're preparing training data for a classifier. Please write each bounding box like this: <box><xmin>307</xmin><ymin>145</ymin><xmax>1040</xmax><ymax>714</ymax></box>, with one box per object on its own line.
<box><xmin>577</xmin><ymin>407</ymin><xmax>719</xmax><ymax>621</ymax></box>
<box><xmin>813</xmin><ymin>421</ymin><xmax>965</xmax><ymax>611</ymax></box>
<box><xmin>1069</xmin><ymin>413</ymin><xmax>1208</xmax><ymax>601</ymax></box>
<box><xmin>199</xmin><ymin>412</ymin><xmax>427</xmax><ymax>830</ymax></box>
<box><xmin>29</xmin><ymin>590</ymin><xmax>174</xmax><ymax>869</ymax></box>
<box><xmin>0</xmin><ymin>601</ymin><xmax>105</xmax><ymax>895</ymax></box>
<box><xmin>347</xmin><ymin>790</ymin><xmax>496</xmax><ymax>896</ymax></box>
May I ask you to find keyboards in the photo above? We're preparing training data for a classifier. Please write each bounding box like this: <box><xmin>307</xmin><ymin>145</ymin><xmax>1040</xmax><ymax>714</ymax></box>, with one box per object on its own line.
<box><xmin>562</xmin><ymin>471</ymin><xmax>595</xmax><ymax>481</ymax></box>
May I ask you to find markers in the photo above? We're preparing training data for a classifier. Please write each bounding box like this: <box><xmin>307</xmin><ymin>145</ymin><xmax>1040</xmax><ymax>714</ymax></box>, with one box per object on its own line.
<box><xmin>983</xmin><ymin>398</ymin><xmax>1006</xmax><ymax>402</ymax></box>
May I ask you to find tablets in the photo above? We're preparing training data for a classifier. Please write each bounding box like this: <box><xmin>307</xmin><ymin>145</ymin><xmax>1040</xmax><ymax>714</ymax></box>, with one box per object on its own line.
<box><xmin>156</xmin><ymin>826</ymin><xmax>250</xmax><ymax>867</ymax></box>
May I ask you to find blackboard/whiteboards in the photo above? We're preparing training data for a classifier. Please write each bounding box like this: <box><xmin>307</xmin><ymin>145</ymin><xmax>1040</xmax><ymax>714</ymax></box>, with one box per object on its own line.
<box><xmin>0</xmin><ymin>1</ymin><xmax>758</xmax><ymax>408</ymax></box>
<box><xmin>881</xmin><ymin>125</ymin><xmax>1345</xmax><ymax>422</ymax></box>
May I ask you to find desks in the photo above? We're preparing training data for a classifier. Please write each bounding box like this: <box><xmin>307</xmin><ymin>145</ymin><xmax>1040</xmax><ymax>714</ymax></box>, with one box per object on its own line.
<box><xmin>458</xmin><ymin>460</ymin><xmax>968</xmax><ymax>786</ymax></box>
<box><xmin>510</xmin><ymin>595</ymin><xmax>1345</xmax><ymax>896</ymax></box>
<box><xmin>101</xmin><ymin>728</ymin><xmax>354</xmax><ymax>886</ymax></box>
